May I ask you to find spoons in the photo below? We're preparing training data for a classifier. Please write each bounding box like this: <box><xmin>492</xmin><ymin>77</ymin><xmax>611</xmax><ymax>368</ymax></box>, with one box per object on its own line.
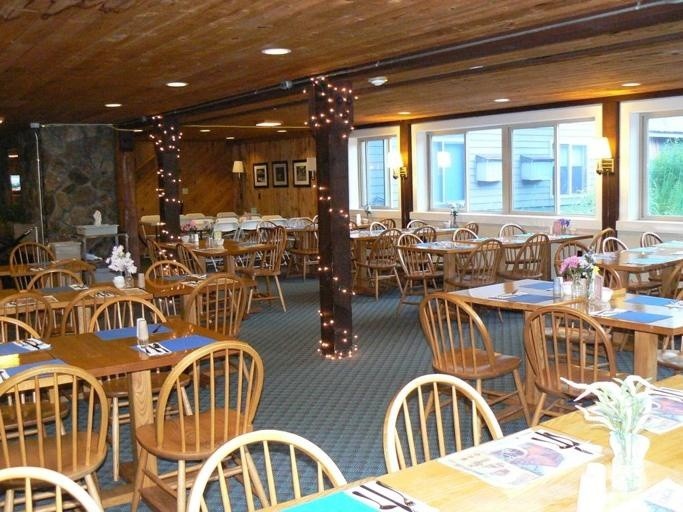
<box><xmin>351</xmin><ymin>490</ymin><xmax>397</xmax><ymax>510</ymax></box>
<box><xmin>529</xmin><ymin>436</ymin><xmax>571</xmax><ymax>450</ymax></box>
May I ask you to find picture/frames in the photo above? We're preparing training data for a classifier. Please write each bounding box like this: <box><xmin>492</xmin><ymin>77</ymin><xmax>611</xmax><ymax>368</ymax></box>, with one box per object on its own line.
<box><xmin>251</xmin><ymin>163</ymin><xmax>269</xmax><ymax>188</ymax></box>
<box><xmin>291</xmin><ymin>159</ymin><xmax>311</xmax><ymax>188</ymax></box>
<box><xmin>271</xmin><ymin>160</ymin><xmax>289</xmax><ymax>188</ymax></box>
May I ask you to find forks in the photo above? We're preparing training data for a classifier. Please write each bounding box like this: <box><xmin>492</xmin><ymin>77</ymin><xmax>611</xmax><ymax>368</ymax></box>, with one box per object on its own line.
<box><xmin>543</xmin><ymin>430</ymin><xmax>581</xmax><ymax>445</ymax></box>
<box><xmin>375</xmin><ymin>478</ymin><xmax>416</xmax><ymax>508</ymax></box>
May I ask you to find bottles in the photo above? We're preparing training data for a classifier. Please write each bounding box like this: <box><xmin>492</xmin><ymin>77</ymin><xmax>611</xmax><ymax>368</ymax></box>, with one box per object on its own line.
<box><xmin>136</xmin><ymin>318</ymin><xmax>149</xmax><ymax>340</ymax></box>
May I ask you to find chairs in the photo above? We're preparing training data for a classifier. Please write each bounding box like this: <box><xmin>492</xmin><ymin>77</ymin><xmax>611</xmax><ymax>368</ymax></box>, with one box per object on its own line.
<box><xmin>524</xmin><ymin>305</ymin><xmax>633</xmax><ymax>433</ymax></box>
<box><xmin>89</xmin><ymin>298</ymin><xmax>193</xmax><ymax>482</ymax></box>
<box><xmin>417</xmin><ymin>296</ymin><xmax>533</xmax><ymax>445</ymax></box>
<box><xmin>0</xmin><ymin>207</ymin><xmax>683</xmax><ymax>334</ymax></box>
<box><xmin>189</xmin><ymin>430</ymin><xmax>351</xmax><ymax>511</ymax></box>
<box><xmin>381</xmin><ymin>373</ymin><xmax>504</xmax><ymax>471</ymax></box>
<box><xmin>0</xmin><ymin>316</ymin><xmax>71</xmax><ymax>442</ymax></box>
<box><xmin>131</xmin><ymin>341</ymin><xmax>264</xmax><ymax>512</ymax></box>
<box><xmin>0</xmin><ymin>364</ymin><xmax>110</xmax><ymax>512</ymax></box>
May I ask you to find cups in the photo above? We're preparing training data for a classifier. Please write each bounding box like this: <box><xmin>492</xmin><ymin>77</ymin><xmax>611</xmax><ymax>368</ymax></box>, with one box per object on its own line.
<box><xmin>355</xmin><ymin>214</ymin><xmax>361</xmax><ymax>226</ymax></box>
<box><xmin>504</xmin><ymin>279</ymin><xmax>514</xmax><ymax>293</ymax></box>
<box><xmin>553</xmin><ymin>277</ymin><xmax>563</xmax><ymax>298</ymax></box>
<box><xmin>137</xmin><ymin>272</ymin><xmax>145</xmax><ymax>288</ymax></box>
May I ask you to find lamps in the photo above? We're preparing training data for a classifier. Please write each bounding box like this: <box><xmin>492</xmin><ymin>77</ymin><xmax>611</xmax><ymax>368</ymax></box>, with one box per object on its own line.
<box><xmin>383</xmin><ymin>144</ymin><xmax>408</xmax><ymax>182</ymax></box>
<box><xmin>232</xmin><ymin>160</ymin><xmax>244</xmax><ymax>179</ymax></box>
<box><xmin>593</xmin><ymin>135</ymin><xmax>614</xmax><ymax>176</ymax></box>
<box><xmin>305</xmin><ymin>157</ymin><xmax>316</xmax><ymax>179</ymax></box>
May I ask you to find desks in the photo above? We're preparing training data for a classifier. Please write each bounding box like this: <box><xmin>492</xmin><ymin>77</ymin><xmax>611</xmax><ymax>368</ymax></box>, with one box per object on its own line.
<box><xmin>0</xmin><ymin>321</ymin><xmax>251</xmax><ymax>500</ymax></box>
<box><xmin>442</xmin><ymin>278</ymin><xmax>683</xmax><ymax>388</ymax></box>
<box><xmin>256</xmin><ymin>375</ymin><xmax>683</xmax><ymax>512</ymax></box>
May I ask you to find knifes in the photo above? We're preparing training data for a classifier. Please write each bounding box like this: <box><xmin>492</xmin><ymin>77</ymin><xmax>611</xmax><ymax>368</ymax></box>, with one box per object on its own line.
<box><xmin>139</xmin><ymin>342</ymin><xmax>167</xmax><ymax>355</ymax></box>
<box><xmin>534</xmin><ymin>430</ymin><xmax>593</xmax><ymax>456</ymax></box>
<box><xmin>360</xmin><ymin>482</ymin><xmax>415</xmax><ymax>512</ymax></box>
<box><xmin>152</xmin><ymin>324</ymin><xmax>161</xmax><ymax>334</ymax></box>
